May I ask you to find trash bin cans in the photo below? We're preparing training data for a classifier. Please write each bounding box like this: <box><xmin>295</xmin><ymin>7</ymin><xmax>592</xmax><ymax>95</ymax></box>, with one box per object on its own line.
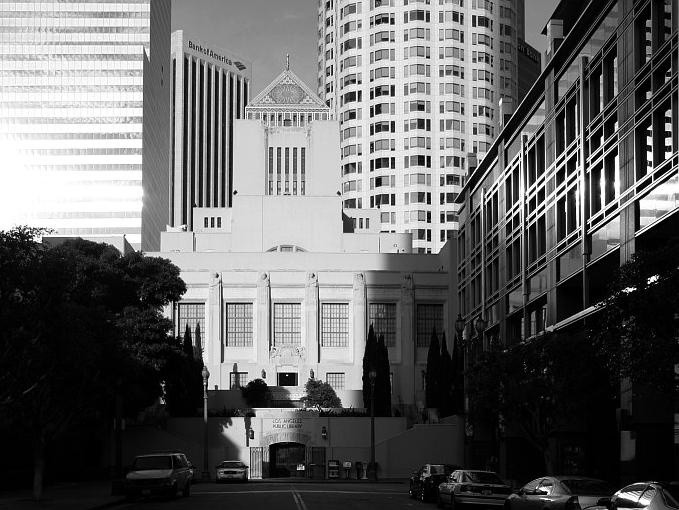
<box><xmin>366</xmin><ymin>462</ymin><xmax>381</xmax><ymax>481</ymax></box>
<box><xmin>328</xmin><ymin>460</ymin><xmax>340</xmax><ymax>480</ymax></box>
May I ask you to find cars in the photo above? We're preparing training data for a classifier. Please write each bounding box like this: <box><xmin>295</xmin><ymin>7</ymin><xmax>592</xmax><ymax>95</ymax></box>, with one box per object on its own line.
<box><xmin>409</xmin><ymin>465</ymin><xmax>679</xmax><ymax>510</ymax></box>
<box><xmin>124</xmin><ymin>451</ymin><xmax>195</xmax><ymax>497</ymax></box>
<box><xmin>215</xmin><ymin>460</ymin><xmax>248</xmax><ymax>483</ymax></box>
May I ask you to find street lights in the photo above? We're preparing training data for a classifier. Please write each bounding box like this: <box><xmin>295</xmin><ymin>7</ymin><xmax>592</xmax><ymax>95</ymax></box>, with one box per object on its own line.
<box><xmin>368</xmin><ymin>366</ymin><xmax>378</xmax><ymax>463</ymax></box>
<box><xmin>453</xmin><ymin>311</ymin><xmax>489</xmax><ymax>413</ymax></box>
<box><xmin>202</xmin><ymin>365</ymin><xmax>210</xmax><ymax>472</ymax></box>
